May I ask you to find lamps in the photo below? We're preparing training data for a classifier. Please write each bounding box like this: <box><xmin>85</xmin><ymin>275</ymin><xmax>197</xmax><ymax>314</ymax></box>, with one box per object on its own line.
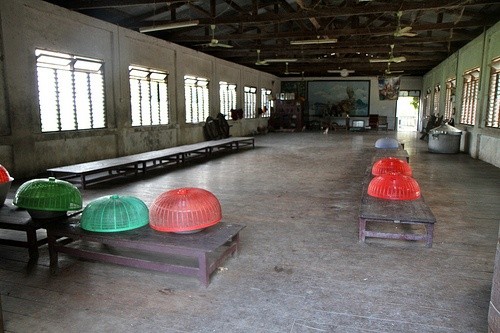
<box><xmin>290</xmin><ymin>38</ymin><xmax>338</xmax><ymax>46</ymax></box>
<box><xmin>139</xmin><ymin>0</ymin><xmax>199</xmax><ymax>32</ymax></box>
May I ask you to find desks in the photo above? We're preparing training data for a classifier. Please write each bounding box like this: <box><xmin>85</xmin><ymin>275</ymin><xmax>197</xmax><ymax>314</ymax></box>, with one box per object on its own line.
<box><xmin>45</xmin><ymin>215</ymin><xmax>246</xmax><ymax>287</ymax></box>
<box><xmin>46</xmin><ymin>137</ymin><xmax>255</xmax><ymax>189</ymax></box>
<box><xmin>357</xmin><ymin>143</ymin><xmax>437</xmax><ymax>248</ymax></box>
<box><xmin>0</xmin><ymin>198</ymin><xmax>83</xmax><ymax>261</ymax></box>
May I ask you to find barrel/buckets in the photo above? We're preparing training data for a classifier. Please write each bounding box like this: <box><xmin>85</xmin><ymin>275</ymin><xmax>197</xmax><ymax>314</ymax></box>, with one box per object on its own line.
<box><xmin>427</xmin><ymin>128</ymin><xmax>462</xmax><ymax>154</ymax></box>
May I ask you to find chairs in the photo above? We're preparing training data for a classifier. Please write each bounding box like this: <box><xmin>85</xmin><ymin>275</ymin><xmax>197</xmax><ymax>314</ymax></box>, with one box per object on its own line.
<box><xmin>369</xmin><ymin>114</ymin><xmax>388</xmax><ymax>132</ymax></box>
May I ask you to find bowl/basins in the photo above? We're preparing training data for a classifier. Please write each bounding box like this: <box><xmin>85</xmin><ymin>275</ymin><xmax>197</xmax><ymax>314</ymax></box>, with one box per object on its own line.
<box><xmin>17</xmin><ymin>185</ymin><xmax>77</xmax><ymax>219</ymax></box>
<box><xmin>154</xmin><ymin>200</ymin><xmax>207</xmax><ymax>234</ymax></box>
<box><xmin>0</xmin><ymin>177</ymin><xmax>15</xmax><ymax>207</ymax></box>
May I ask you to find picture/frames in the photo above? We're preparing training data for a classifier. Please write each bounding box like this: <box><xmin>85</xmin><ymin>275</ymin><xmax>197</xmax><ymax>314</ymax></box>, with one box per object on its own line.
<box><xmin>307</xmin><ymin>80</ymin><xmax>370</xmax><ymax>117</ymax></box>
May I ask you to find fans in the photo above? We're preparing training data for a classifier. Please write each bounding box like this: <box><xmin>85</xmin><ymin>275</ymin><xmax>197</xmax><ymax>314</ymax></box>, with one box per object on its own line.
<box><xmin>369</xmin><ymin>11</ymin><xmax>416</xmax><ymax>74</ymax></box>
<box><xmin>283</xmin><ymin>62</ymin><xmax>300</xmax><ymax>74</ymax></box>
<box><xmin>245</xmin><ymin>50</ymin><xmax>269</xmax><ymax>66</ymax></box>
<box><xmin>192</xmin><ymin>25</ymin><xmax>234</xmax><ymax>48</ymax></box>
